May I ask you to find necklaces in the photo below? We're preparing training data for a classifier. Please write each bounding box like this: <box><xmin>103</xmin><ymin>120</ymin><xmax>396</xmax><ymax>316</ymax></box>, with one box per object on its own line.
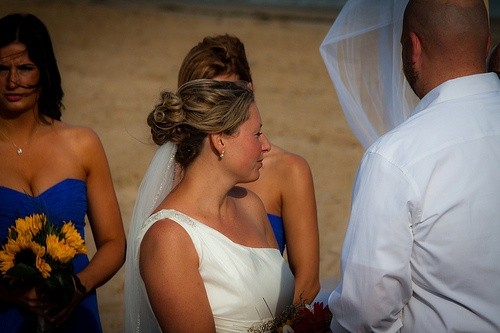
<box><xmin>0</xmin><ymin>117</ymin><xmax>44</xmax><ymax>155</ymax></box>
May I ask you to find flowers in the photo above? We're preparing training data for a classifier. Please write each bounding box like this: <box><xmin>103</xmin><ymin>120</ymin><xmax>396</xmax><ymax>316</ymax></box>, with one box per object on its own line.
<box><xmin>273</xmin><ymin>289</ymin><xmax>334</xmax><ymax>333</ymax></box>
<box><xmin>0</xmin><ymin>211</ymin><xmax>88</xmax><ymax>333</ymax></box>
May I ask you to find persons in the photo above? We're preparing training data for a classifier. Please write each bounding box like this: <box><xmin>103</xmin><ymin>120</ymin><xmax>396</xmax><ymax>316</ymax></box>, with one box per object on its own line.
<box><xmin>133</xmin><ymin>78</ymin><xmax>297</xmax><ymax>333</ymax></box>
<box><xmin>168</xmin><ymin>33</ymin><xmax>321</xmax><ymax>313</ymax></box>
<box><xmin>329</xmin><ymin>1</ymin><xmax>500</xmax><ymax>332</ymax></box>
<box><xmin>0</xmin><ymin>13</ymin><xmax>126</xmax><ymax>333</ymax></box>
